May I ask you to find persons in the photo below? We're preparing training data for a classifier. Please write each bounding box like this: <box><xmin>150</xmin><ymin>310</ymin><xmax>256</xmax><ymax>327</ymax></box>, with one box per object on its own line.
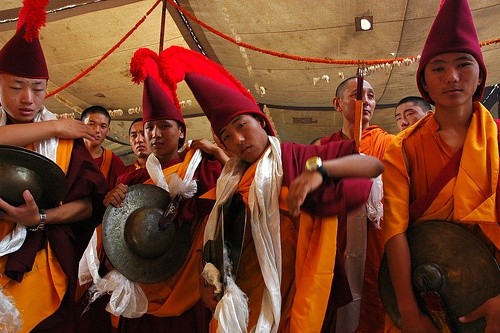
<box><xmin>0</xmin><ymin>0</ymin><xmax>108</xmax><ymax>333</ymax></box>
<box><xmin>161</xmin><ymin>46</ymin><xmax>386</xmax><ymax>333</ymax></box>
<box><xmin>99</xmin><ymin>47</ymin><xmax>224</xmax><ymax>333</ymax></box>
<box><xmin>370</xmin><ymin>0</ymin><xmax>500</xmax><ymax>333</ymax></box>
<box><xmin>190</xmin><ymin>124</ymin><xmax>233</xmax><ymax>167</ymax></box>
<box><xmin>317</xmin><ymin>77</ymin><xmax>398</xmax><ymax>333</ymax></box>
<box><xmin>310</xmin><ymin>137</ymin><xmax>325</xmax><ymax>146</ymax></box>
<box><xmin>124</xmin><ymin>118</ymin><xmax>150</xmax><ymax>170</ymax></box>
<box><xmin>395</xmin><ymin>96</ymin><xmax>434</xmax><ymax>133</ymax></box>
<box><xmin>77</xmin><ymin>105</ymin><xmax>126</xmax><ymax>190</ymax></box>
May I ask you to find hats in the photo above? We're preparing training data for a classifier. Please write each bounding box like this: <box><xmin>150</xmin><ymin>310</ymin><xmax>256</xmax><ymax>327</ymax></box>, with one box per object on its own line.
<box><xmin>129</xmin><ymin>47</ymin><xmax>187</xmax><ymax>150</ymax></box>
<box><xmin>158</xmin><ymin>45</ymin><xmax>275</xmax><ymax>148</ymax></box>
<box><xmin>416</xmin><ymin>0</ymin><xmax>487</xmax><ymax>106</ymax></box>
<box><xmin>0</xmin><ymin>0</ymin><xmax>50</xmax><ymax>81</ymax></box>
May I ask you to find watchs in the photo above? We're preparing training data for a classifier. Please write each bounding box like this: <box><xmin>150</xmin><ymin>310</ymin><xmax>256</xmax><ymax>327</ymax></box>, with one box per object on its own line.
<box><xmin>28</xmin><ymin>211</ymin><xmax>46</xmax><ymax>232</ymax></box>
<box><xmin>306</xmin><ymin>156</ymin><xmax>326</xmax><ymax>190</ymax></box>
